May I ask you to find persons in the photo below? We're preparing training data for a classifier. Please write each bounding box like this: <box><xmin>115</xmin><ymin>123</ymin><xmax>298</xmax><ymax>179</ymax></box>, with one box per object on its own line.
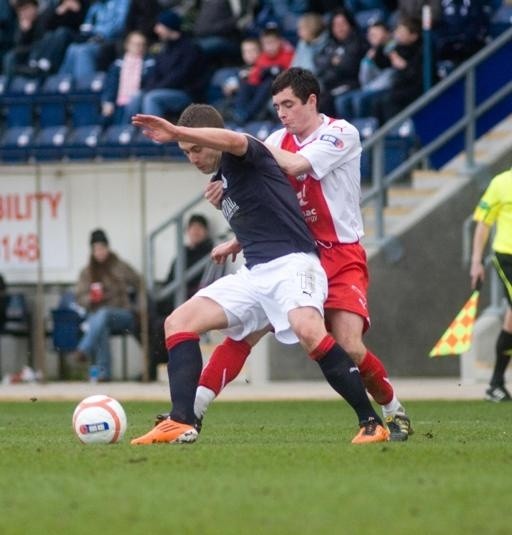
<box><xmin>131</xmin><ymin>104</ymin><xmax>389</xmax><ymax>444</ymax></box>
<box><xmin>156</xmin><ymin>68</ymin><xmax>414</xmax><ymax>442</ymax></box>
<box><xmin>470</xmin><ymin>166</ymin><xmax>512</xmax><ymax>402</ymax></box>
<box><xmin>73</xmin><ymin>229</ymin><xmax>140</xmax><ymax>382</ymax></box>
<box><xmin>148</xmin><ymin>215</ymin><xmax>226</xmax><ymax>379</ymax></box>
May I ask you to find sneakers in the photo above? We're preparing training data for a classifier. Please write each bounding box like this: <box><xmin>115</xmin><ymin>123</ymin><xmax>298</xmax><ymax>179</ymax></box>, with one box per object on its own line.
<box><xmin>154</xmin><ymin>412</ymin><xmax>203</xmax><ymax>435</ymax></box>
<box><xmin>130</xmin><ymin>417</ymin><xmax>200</xmax><ymax>446</ymax></box>
<box><xmin>72</xmin><ymin>349</ymin><xmax>112</xmax><ymax>382</ymax></box>
<box><xmin>351</xmin><ymin>423</ymin><xmax>390</xmax><ymax>446</ymax></box>
<box><xmin>484</xmin><ymin>383</ymin><xmax>511</xmax><ymax>406</ymax></box>
<box><xmin>384</xmin><ymin>411</ymin><xmax>414</xmax><ymax>443</ymax></box>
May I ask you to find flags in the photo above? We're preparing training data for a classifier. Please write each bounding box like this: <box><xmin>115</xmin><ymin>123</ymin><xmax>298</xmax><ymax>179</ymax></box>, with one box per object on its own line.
<box><xmin>429</xmin><ymin>278</ymin><xmax>482</xmax><ymax>358</ymax></box>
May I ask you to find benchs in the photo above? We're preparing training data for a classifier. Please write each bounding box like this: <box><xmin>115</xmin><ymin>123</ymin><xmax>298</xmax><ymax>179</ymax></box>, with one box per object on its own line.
<box><xmin>0</xmin><ymin>292</ymin><xmax>34</xmax><ymax>373</ymax></box>
<box><xmin>57</xmin><ymin>281</ymin><xmax>165</xmax><ymax>381</ymax></box>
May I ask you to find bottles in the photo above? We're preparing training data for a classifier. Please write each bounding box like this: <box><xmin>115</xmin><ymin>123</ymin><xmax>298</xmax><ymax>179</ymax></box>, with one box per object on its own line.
<box><xmin>88</xmin><ymin>366</ymin><xmax>101</xmax><ymax>385</ymax></box>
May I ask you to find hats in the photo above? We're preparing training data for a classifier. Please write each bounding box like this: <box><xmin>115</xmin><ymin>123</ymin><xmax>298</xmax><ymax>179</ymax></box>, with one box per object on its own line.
<box><xmin>90</xmin><ymin>230</ymin><xmax>107</xmax><ymax>246</ymax></box>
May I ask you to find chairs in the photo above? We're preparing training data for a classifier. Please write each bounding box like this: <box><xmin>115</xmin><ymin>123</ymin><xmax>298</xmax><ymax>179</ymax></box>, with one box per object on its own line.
<box><xmin>0</xmin><ymin>0</ymin><xmax>420</xmax><ymax>182</ymax></box>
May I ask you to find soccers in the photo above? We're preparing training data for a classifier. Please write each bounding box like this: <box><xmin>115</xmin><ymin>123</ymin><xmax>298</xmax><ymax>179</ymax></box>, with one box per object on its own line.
<box><xmin>72</xmin><ymin>394</ymin><xmax>127</xmax><ymax>444</ymax></box>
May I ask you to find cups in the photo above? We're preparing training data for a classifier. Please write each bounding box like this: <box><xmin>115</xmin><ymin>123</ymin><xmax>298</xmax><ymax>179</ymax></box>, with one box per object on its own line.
<box><xmin>90</xmin><ymin>282</ymin><xmax>104</xmax><ymax>305</ymax></box>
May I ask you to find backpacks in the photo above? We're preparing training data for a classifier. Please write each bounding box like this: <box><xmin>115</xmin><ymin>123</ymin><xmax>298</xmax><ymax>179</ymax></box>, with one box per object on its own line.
<box><xmin>50</xmin><ymin>303</ymin><xmax>83</xmax><ymax>354</ymax></box>
